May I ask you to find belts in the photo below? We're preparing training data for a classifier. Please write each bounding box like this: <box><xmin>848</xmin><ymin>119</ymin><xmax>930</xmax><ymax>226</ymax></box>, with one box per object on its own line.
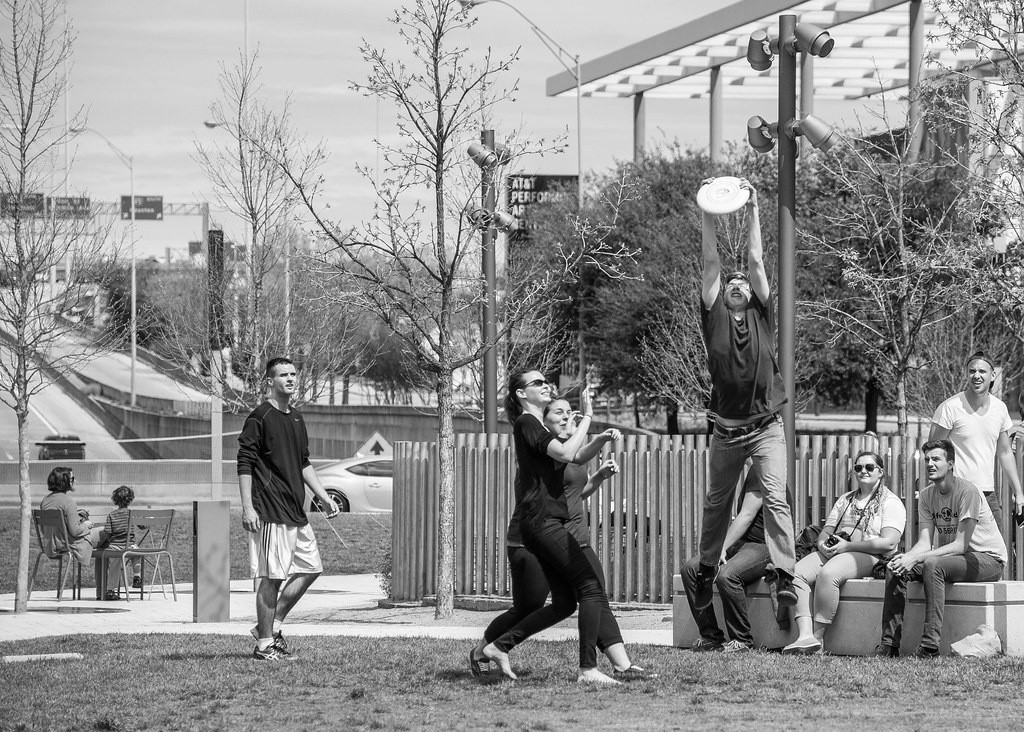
<box><xmin>714</xmin><ymin>414</ymin><xmax>776</xmax><ymax>437</ymax></box>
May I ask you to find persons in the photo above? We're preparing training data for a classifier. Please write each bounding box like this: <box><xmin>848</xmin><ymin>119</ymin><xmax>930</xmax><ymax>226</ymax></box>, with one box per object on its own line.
<box><xmin>470</xmin><ymin>366</ymin><xmax>657</xmax><ymax>685</ymax></box>
<box><xmin>236</xmin><ymin>357</ymin><xmax>340</xmax><ymax>662</ymax></box>
<box><xmin>692</xmin><ymin>176</ymin><xmax>799</xmax><ymax>611</ymax></box>
<box><xmin>680</xmin><ymin>455</ymin><xmax>793</xmax><ymax>654</ymax></box>
<box><xmin>928</xmin><ymin>350</ymin><xmax>1024</xmax><ymax>543</ymax></box>
<box><xmin>781</xmin><ymin>452</ymin><xmax>906</xmax><ymax>656</ymax></box>
<box><xmin>104</xmin><ymin>486</ymin><xmax>141</xmax><ymax>596</ymax></box>
<box><xmin>859</xmin><ymin>439</ymin><xmax>1008</xmax><ymax>659</ymax></box>
<box><xmin>40</xmin><ymin>467</ymin><xmax>121</xmax><ymax>600</ymax></box>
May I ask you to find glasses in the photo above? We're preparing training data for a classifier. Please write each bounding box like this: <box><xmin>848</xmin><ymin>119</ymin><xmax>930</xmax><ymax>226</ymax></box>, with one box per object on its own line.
<box><xmin>726</xmin><ymin>275</ymin><xmax>743</xmax><ymax>279</ymax></box>
<box><xmin>920</xmin><ymin>440</ymin><xmax>947</xmax><ymax>451</ymax></box>
<box><xmin>525</xmin><ymin>379</ymin><xmax>550</xmax><ymax>386</ymax></box>
<box><xmin>855</xmin><ymin>464</ymin><xmax>882</xmax><ymax>473</ymax></box>
<box><xmin>70</xmin><ymin>476</ymin><xmax>75</xmax><ymax>481</ymax></box>
<box><xmin>725</xmin><ymin>283</ymin><xmax>750</xmax><ymax>290</ymax></box>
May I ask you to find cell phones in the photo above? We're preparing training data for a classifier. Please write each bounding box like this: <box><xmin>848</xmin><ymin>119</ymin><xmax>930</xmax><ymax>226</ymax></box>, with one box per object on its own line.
<box><xmin>1014</xmin><ymin>506</ymin><xmax>1024</xmax><ymax>527</ymax></box>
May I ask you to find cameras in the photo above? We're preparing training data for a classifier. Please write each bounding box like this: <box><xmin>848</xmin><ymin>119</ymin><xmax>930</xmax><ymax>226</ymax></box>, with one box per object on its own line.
<box><xmin>827</xmin><ymin>531</ymin><xmax>851</xmax><ymax>547</ymax></box>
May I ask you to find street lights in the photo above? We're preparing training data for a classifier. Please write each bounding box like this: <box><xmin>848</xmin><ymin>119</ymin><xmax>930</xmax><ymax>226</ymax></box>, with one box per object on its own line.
<box><xmin>745</xmin><ymin>10</ymin><xmax>841</xmax><ymax>542</ymax></box>
<box><xmin>459</xmin><ymin>0</ymin><xmax>585</xmax><ymax>213</ymax></box>
<box><xmin>464</xmin><ymin>129</ymin><xmax>520</xmax><ymax>434</ymax></box>
<box><xmin>203</xmin><ymin>119</ymin><xmax>291</xmax><ymax>362</ymax></box>
<box><xmin>69</xmin><ymin>125</ymin><xmax>140</xmax><ymax>408</ymax></box>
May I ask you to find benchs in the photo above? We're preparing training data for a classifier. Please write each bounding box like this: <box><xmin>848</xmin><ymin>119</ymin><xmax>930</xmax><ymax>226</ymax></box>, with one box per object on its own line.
<box><xmin>673</xmin><ymin>574</ymin><xmax>1024</xmax><ymax>656</ymax></box>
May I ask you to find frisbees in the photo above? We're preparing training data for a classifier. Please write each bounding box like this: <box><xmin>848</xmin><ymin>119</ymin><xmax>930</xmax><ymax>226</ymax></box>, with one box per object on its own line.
<box><xmin>696</xmin><ymin>175</ymin><xmax>751</xmax><ymax>215</ymax></box>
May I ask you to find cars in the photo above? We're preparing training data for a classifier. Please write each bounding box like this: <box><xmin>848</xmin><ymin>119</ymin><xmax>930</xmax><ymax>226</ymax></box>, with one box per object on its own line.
<box><xmin>300</xmin><ymin>454</ymin><xmax>397</xmax><ymax>512</ymax></box>
<box><xmin>35</xmin><ymin>434</ymin><xmax>87</xmax><ymax>459</ymax></box>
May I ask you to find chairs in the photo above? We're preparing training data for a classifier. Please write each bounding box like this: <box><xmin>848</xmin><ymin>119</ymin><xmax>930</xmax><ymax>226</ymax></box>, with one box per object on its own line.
<box><xmin>27</xmin><ymin>509</ymin><xmax>177</xmax><ymax>602</ymax></box>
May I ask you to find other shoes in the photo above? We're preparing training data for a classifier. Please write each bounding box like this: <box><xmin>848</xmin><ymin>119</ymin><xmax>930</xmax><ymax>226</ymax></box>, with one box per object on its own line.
<box><xmin>868</xmin><ymin>647</ymin><xmax>900</xmax><ymax>658</ymax></box>
<box><xmin>910</xmin><ymin>647</ymin><xmax>941</xmax><ymax>658</ymax></box>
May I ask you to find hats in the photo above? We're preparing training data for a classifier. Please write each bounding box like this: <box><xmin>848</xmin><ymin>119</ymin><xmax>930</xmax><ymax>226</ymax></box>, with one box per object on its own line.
<box><xmin>723</xmin><ymin>272</ymin><xmax>749</xmax><ymax>281</ymax></box>
<box><xmin>967</xmin><ymin>350</ymin><xmax>995</xmax><ymax>388</ymax></box>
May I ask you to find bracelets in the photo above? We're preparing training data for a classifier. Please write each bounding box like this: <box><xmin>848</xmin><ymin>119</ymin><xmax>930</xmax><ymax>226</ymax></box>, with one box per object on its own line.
<box><xmin>584</xmin><ymin>413</ymin><xmax>592</xmax><ymax>420</ymax></box>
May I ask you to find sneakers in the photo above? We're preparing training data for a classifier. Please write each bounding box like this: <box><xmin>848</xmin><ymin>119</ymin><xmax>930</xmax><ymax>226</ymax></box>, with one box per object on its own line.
<box><xmin>690</xmin><ymin>637</ymin><xmax>726</xmax><ymax>650</ymax></box>
<box><xmin>106</xmin><ymin>591</ymin><xmax>121</xmax><ymax>600</ymax></box>
<box><xmin>614</xmin><ymin>664</ymin><xmax>658</xmax><ymax>680</ymax></box>
<box><xmin>470</xmin><ymin>646</ymin><xmax>490</xmax><ymax>676</ymax></box>
<box><xmin>255</xmin><ymin>643</ymin><xmax>290</xmax><ymax>660</ymax></box>
<box><xmin>132</xmin><ymin>576</ymin><xmax>143</xmax><ymax>588</ymax></box>
<box><xmin>251</xmin><ymin>624</ymin><xmax>288</xmax><ymax>650</ymax></box>
<box><xmin>776</xmin><ymin>580</ymin><xmax>805</xmax><ymax>606</ymax></box>
<box><xmin>694</xmin><ymin>564</ymin><xmax>720</xmax><ymax>609</ymax></box>
<box><xmin>723</xmin><ymin>641</ymin><xmax>753</xmax><ymax>652</ymax></box>
<box><xmin>783</xmin><ymin>637</ymin><xmax>820</xmax><ymax>653</ymax></box>
